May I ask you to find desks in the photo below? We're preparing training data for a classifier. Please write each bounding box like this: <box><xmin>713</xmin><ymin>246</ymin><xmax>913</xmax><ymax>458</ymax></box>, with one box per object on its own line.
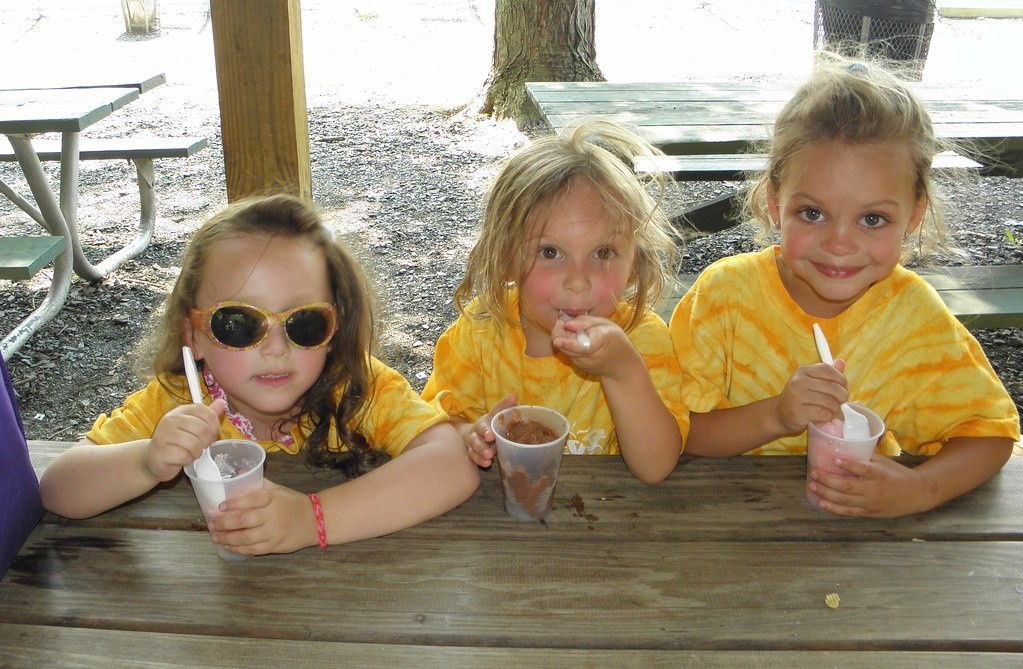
<box><xmin>0</xmin><ymin>71</ymin><xmax>165</xmax><ymax>236</ymax></box>
<box><xmin>1</xmin><ymin>441</ymin><xmax>1023</xmax><ymax>669</ymax></box>
<box><xmin>525</xmin><ymin>79</ymin><xmax>1023</xmax><ymax>164</ymax></box>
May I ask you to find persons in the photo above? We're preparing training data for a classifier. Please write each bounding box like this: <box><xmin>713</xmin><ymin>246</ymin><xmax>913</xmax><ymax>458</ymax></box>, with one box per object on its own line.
<box><xmin>37</xmin><ymin>194</ymin><xmax>481</xmax><ymax>555</ymax></box>
<box><xmin>420</xmin><ymin>120</ymin><xmax>691</xmax><ymax>484</ymax></box>
<box><xmin>669</xmin><ymin>63</ymin><xmax>1020</xmax><ymax>518</ymax></box>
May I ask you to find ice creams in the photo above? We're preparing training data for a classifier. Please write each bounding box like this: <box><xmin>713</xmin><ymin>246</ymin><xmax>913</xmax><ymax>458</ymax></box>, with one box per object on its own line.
<box><xmin>490</xmin><ymin>404</ymin><xmax>571</xmax><ymax>520</ymax></box>
<box><xmin>183</xmin><ymin>438</ymin><xmax>266</xmax><ymax>561</ymax></box>
<box><xmin>806</xmin><ymin>399</ymin><xmax>886</xmax><ymax>510</ymax></box>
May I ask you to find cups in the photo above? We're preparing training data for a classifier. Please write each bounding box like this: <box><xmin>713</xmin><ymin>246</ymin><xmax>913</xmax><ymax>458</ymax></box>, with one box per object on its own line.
<box><xmin>490</xmin><ymin>405</ymin><xmax>570</xmax><ymax>522</ymax></box>
<box><xmin>183</xmin><ymin>439</ymin><xmax>267</xmax><ymax>563</ymax></box>
<box><xmin>807</xmin><ymin>402</ymin><xmax>885</xmax><ymax>512</ymax></box>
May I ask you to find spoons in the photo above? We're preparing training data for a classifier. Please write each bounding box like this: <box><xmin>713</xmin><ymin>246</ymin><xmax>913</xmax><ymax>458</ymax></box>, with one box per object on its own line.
<box><xmin>182</xmin><ymin>346</ymin><xmax>226</xmax><ymax>510</ymax></box>
<box><xmin>557</xmin><ymin>310</ymin><xmax>591</xmax><ymax>351</ymax></box>
<box><xmin>812</xmin><ymin>322</ymin><xmax>872</xmax><ymax>462</ymax></box>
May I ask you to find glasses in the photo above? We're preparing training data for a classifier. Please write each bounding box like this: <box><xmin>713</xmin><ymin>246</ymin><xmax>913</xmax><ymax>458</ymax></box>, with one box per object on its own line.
<box><xmin>188</xmin><ymin>301</ymin><xmax>340</xmax><ymax>351</ymax></box>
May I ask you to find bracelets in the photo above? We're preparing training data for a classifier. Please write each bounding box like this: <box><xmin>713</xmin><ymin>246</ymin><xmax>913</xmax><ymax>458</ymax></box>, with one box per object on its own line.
<box><xmin>309</xmin><ymin>494</ymin><xmax>326</xmax><ymax>549</ymax></box>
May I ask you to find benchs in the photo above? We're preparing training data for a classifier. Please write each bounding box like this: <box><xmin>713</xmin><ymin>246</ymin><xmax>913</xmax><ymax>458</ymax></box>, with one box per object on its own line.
<box><xmin>647</xmin><ymin>149</ymin><xmax>1023</xmax><ymax>329</ymax></box>
<box><xmin>1</xmin><ymin>136</ymin><xmax>208</xmax><ymax>364</ymax></box>
